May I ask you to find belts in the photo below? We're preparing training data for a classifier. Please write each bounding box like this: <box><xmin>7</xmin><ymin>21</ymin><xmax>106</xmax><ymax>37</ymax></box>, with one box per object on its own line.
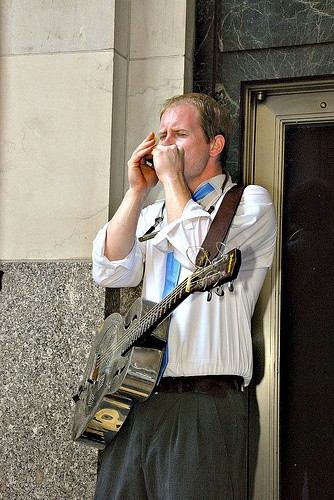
<box><xmin>152</xmin><ymin>375</ymin><xmax>244</xmax><ymax>398</ymax></box>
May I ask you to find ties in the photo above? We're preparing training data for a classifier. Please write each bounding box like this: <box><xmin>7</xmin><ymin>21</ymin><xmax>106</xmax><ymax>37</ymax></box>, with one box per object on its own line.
<box><xmin>156</xmin><ymin>183</ymin><xmax>215</xmax><ymax>386</ymax></box>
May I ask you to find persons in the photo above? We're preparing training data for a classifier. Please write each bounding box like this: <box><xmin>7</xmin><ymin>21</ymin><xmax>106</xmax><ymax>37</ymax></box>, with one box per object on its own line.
<box><xmin>91</xmin><ymin>92</ymin><xmax>277</xmax><ymax>500</ymax></box>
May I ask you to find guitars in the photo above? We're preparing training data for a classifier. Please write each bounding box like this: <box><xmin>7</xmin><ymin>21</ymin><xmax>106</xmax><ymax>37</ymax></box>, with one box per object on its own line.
<box><xmin>73</xmin><ymin>241</ymin><xmax>242</xmax><ymax>450</ymax></box>
